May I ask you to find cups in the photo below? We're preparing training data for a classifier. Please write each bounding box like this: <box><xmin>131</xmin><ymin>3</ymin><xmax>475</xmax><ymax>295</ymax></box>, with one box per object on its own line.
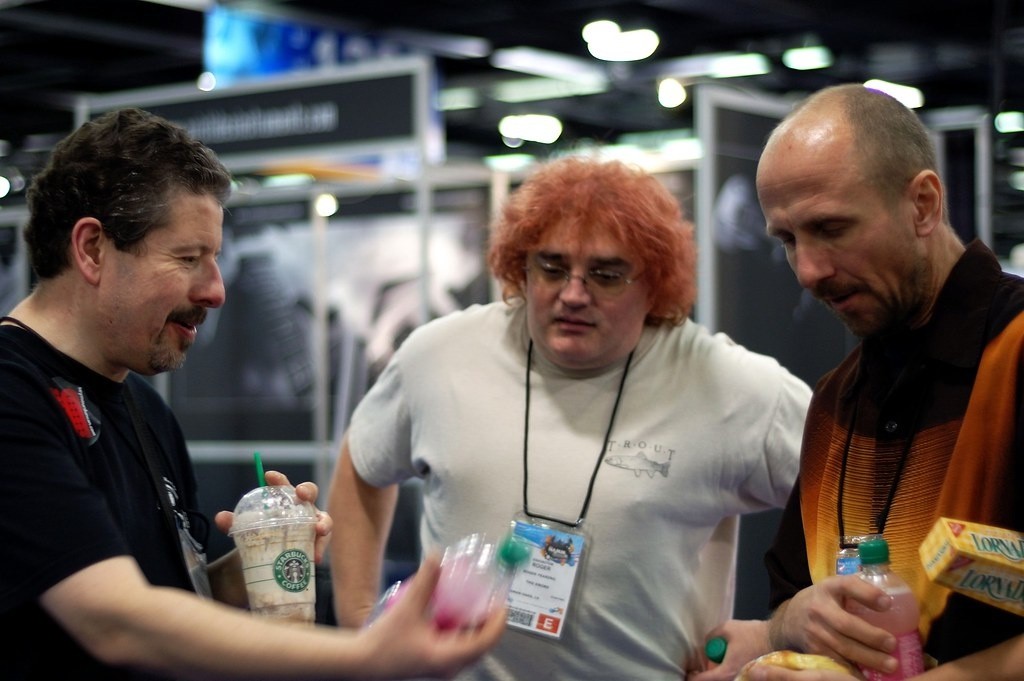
<box><xmin>228</xmin><ymin>483</ymin><xmax>318</xmax><ymax>622</ymax></box>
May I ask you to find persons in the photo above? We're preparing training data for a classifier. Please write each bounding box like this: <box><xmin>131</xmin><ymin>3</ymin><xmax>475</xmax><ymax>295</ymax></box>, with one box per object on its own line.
<box><xmin>0</xmin><ymin>107</ymin><xmax>508</xmax><ymax>681</ymax></box>
<box><xmin>325</xmin><ymin>156</ymin><xmax>814</xmax><ymax>680</ymax></box>
<box><xmin>757</xmin><ymin>85</ymin><xmax>1024</xmax><ymax>680</ymax></box>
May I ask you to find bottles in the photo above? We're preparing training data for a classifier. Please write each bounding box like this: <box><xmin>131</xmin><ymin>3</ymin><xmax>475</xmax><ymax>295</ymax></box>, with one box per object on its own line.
<box><xmin>702</xmin><ymin>637</ymin><xmax>727</xmax><ymax>671</ymax></box>
<box><xmin>362</xmin><ymin>532</ymin><xmax>531</xmax><ymax>634</ymax></box>
<box><xmin>845</xmin><ymin>538</ymin><xmax>925</xmax><ymax>680</ymax></box>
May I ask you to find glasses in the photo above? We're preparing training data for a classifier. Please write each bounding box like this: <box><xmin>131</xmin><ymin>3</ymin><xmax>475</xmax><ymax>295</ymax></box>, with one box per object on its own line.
<box><xmin>149</xmin><ymin>466</ymin><xmax>211</xmax><ymax>555</ymax></box>
<box><xmin>522</xmin><ymin>261</ymin><xmax>644</xmax><ymax>296</ymax></box>
